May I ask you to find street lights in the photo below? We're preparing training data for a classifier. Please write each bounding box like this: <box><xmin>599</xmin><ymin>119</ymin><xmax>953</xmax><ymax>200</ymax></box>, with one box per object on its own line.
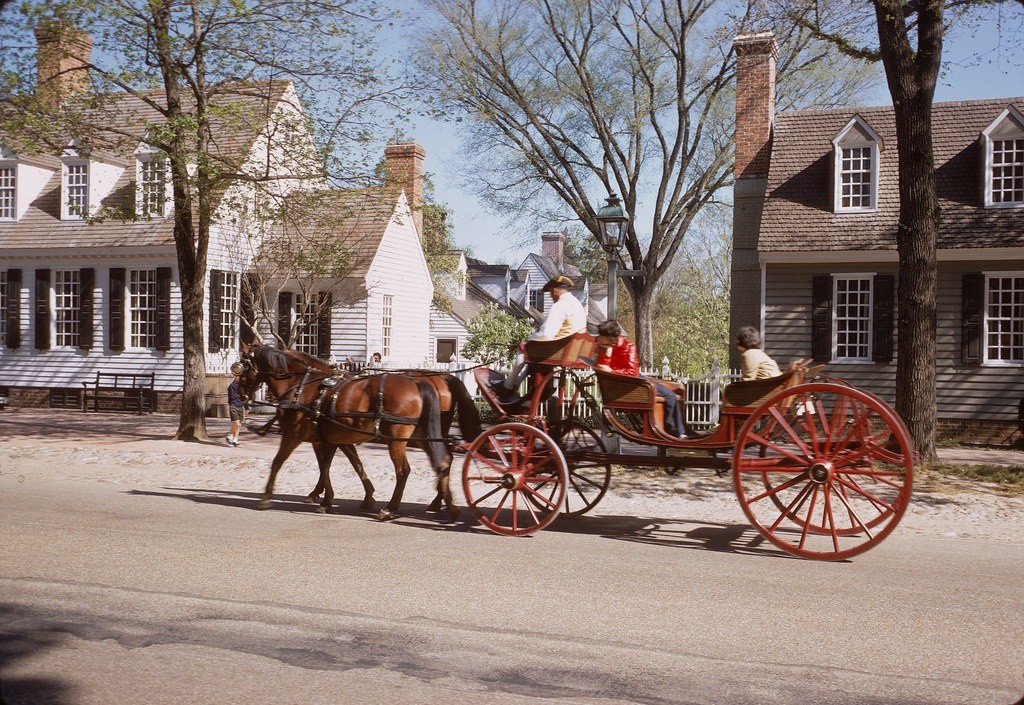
<box><xmin>594</xmin><ymin>189</ymin><xmax>630</xmax><ymax>320</ymax></box>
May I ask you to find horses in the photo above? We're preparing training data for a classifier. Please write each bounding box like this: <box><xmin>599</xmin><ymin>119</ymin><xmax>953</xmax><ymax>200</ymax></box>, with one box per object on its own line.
<box><xmin>236</xmin><ymin>336</ymin><xmax>481</xmax><ymax>524</ymax></box>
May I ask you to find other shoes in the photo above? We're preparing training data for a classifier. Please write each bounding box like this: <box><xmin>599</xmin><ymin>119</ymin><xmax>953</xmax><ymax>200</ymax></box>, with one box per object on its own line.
<box><xmin>678</xmin><ymin>423</ymin><xmax>722</xmax><ymax>440</ymax></box>
<box><xmin>490</xmin><ymin>380</ymin><xmax>516</xmax><ymax>400</ymax></box>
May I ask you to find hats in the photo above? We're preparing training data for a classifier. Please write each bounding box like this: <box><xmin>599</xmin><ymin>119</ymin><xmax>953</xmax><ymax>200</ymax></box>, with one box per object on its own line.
<box><xmin>542</xmin><ymin>275</ymin><xmax>574</xmax><ymax>291</ymax></box>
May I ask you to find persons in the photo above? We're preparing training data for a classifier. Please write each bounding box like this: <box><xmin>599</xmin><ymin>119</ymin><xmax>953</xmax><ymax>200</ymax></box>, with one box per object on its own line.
<box><xmin>716</xmin><ymin>325</ymin><xmax>784</xmax><ymax>424</ymax></box>
<box><xmin>490</xmin><ymin>274</ymin><xmax>586</xmax><ymax>398</ymax></box>
<box><xmin>225</xmin><ymin>376</ymin><xmax>249</xmax><ymax>447</ymax></box>
<box><xmin>593</xmin><ymin>318</ymin><xmax>695</xmax><ymax>440</ymax></box>
<box><xmin>344</xmin><ymin>351</ymin><xmax>385</xmax><ymax>376</ymax></box>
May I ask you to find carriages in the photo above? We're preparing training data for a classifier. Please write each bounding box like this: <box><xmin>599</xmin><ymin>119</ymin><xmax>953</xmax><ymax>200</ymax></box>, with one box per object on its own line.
<box><xmin>231</xmin><ymin>332</ymin><xmax>917</xmax><ymax>565</ymax></box>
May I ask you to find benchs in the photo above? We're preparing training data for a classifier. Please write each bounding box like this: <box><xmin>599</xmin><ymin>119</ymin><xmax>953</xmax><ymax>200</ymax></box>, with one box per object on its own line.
<box><xmin>82</xmin><ymin>371</ymin><xmax>154</xmax><ymax>416</ymax></box>
<box><xmin>526</xmin><ymin>334</ymin><xmax>595</xmax><ymax>368</ymax></box>
<box><xmin>598</xmin><ymin>370</ymin><xmax>684</xmax><ymax>408</ymax></box>
<box><xmin>724</xmin><ymin>359</ymin><xmax>812</xmax><ymax>415</ymax></box>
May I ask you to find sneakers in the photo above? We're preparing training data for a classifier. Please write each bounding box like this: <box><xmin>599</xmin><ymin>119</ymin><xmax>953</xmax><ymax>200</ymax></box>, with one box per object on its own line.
<box><xmin>225</xmin><ymin>437</ymin><xmax>233</xmax><ymax>447</ymax></box>
<box><xmin>232</xmin><ymin>440</ymin><xmax>242</xmax><ymax>448</ymax></box>
